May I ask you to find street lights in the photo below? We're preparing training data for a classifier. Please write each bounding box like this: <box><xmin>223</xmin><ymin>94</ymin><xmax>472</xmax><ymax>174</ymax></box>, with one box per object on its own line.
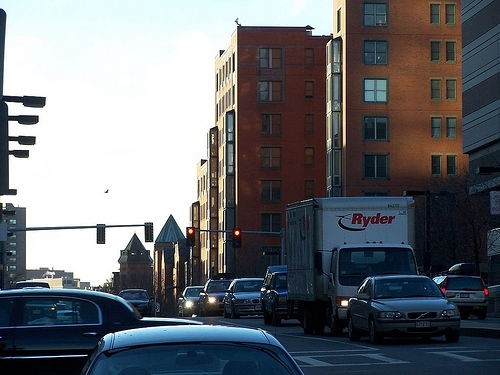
<box><xmin>0</xmin><ymin>202</ymin><xmax>17</xmax><ymax>290</ymax></box>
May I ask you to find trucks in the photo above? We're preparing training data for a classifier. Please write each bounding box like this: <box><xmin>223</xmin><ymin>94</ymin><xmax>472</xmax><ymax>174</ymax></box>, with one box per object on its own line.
<box><xmin>285</xmin><ymin>196</ymin><xmax>432</xmax><ymax>336</ymax></box>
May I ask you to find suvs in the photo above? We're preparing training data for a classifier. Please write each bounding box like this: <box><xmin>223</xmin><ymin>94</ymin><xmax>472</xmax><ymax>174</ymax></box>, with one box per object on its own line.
<box><xmin>260</xmin><ymin>265</ymin><xmax>298</xmax><ymax>325</ymax></box>
<box><xmin>426</xmin><ymin>270</ymin><xmax>490</xmax><ymax>320</ymax></box>
<box><xmin>198</xmin><ymin>278</ymin><xmax>233</xmax><ymax>317</ymax></box>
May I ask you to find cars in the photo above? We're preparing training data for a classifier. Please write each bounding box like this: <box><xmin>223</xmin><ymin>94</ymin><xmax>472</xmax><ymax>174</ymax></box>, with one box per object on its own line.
<box><xmin>222</xmin><ymin>277</ymin><xmax>264</xmax><ymax>319</ymax></box>
<box><xmin>346</xmin><ymin>275</ymin><xmax>461</xmax><ymax>345</ymax></box>
<box><xmin>178</xmin><ymin>286</ymin><xmax>205</xmax><ymax>317</ymax></box>
<box><xmin>80</xmin><ymin>325</ymin><xmax>304</xmax><ymax>374</ymax></box>
<box><xmin>15</xmin><ymin>278</ymin><xmax>80</xmax><ymax>321</ymax></box>
<box><xmin>118</xmin><ymin>289</ymin><xmax>154</xmax><ymax>317</ymax></box>
<box><xmin>0</xmin><ymin>285</ymin><xmax>204</xmax><ymax>375</ymax></box>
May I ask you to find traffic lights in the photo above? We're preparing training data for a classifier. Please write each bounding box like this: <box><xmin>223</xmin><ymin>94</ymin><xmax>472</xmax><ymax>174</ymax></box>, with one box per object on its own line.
<box><xmin>186</xmin><ymin>226</ymin><xmax>196</xmax><ymax>247</ymax></box>
<box><xmin>232</xmin><ymin>228</ymin><xmax>242</xmax><ymax>249</ymax></box>
